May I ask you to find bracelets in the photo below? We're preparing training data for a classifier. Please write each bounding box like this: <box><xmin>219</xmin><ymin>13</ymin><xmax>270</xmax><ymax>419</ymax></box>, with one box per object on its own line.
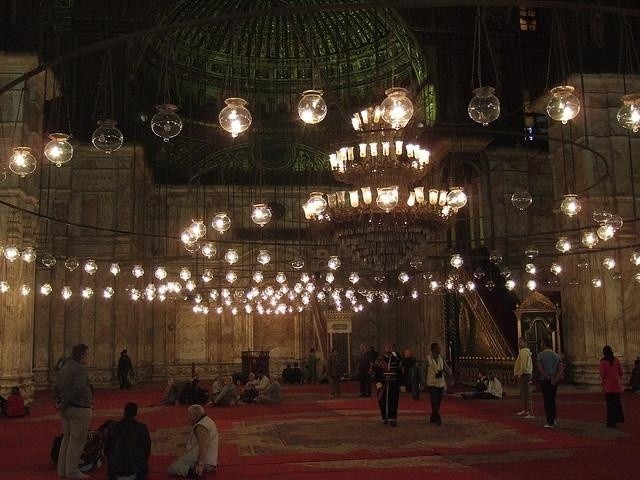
<box><xmin>196</xmin><ymin>462</ymin><xmax>204</xmax><ymax>466</ymax></box>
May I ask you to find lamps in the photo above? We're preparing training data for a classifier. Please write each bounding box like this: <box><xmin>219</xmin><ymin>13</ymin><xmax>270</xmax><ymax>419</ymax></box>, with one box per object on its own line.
<box><xmin>218</xmin><ymin>97</ymin><xmax>253</xmax><ymax>138</ymax></box>
<box><xmin>149</xmin><ymin>104</ymin><xmax>183</xmax><ymax>143</ymax></box>
<box><xmin>580</xmin><ymin>231</ymin><xmax>600</xmax><ymax>250</ymax></box>
<box><xmin>0</xmin><ymin>243</ymin><xmax>183</xmax><ymax>303</ymax></box>
<box><xmin>211</xmin><ymin>211</ymin><xmax>233</xmax><ymax>236</ymax></box>
<box><xmin>549</xmin><ymin>251</ymin><xmax>640</xmax><ymax>290</ymax></box>
<box><xmin>616</xmin><ymin>92</ymin><xmax>640</xmax><ymax>134</ymax></box>
<box><xmin>554</xmin><ymin>235</ymin><xmax>572</xmax><ymax>254</ymax></box>
<box><xmin>183</xmin><ymin>265</ymin><xmax>365</xmax><ymax>319</ymax></box>
<box><xmin>43</xmin><ymin>131</ymin><xmax>74</xmax><ymax>168</ymax></box>
<box><xmin>202</xmin><ymin>242</ymin><xmax>271</xmax><ymax>266</ymax></box>
<box><xmin>329</xmin><ymin>140</ymin><xmax>431</xmax><ymax>173</ymax></box>
<box><xmin>180</xmin><ymin>218</ymin><xmax>208</xmax><ymax>254</ymax></box>
<box><xmin>327</xmin><ymin>255</ymin><xmax>341</xmax><ymax>271</ymax></box>
<box><xmin>379</xmin><ymin>86</ymin><xmax>415</xmax><ymax>128</ymax></box>
<box><xmin>291</xmin><ymin>256</ymin><xmax>305</xmax><ymax>270</ymax></box>
<box><xmin>302</xmin><ymin>185</ymin><xmax>468</xmax><ymax>222</ymax></box>
<box><xmin>592</xmin><ymin>206</ymin><xmax>624</xmax><ymax>242</ymax></box>
<box><xmin>545</xmin><ymin>84</ymin><xmax>582</xmax><ymax>125</ymax></box>
<box><xmin>8</xmin><ymin>145</ymin><xmax>38</xmax><ymax>179</ymax></box>
<box><xmin>366</xmin><ymin>245</ymin><xmax>541</xmax><ymax>306</ymax></box>
<box><xmin>466</xmin><ymin>85</ymin><xmax>502</xmax><ymax>127</ymax></box>
<box><xmin>511</xmin><ymin>190</ymin><xmax>533</xmax><ymax>210</ymax></box>
<box><xmin>297</xmin><ymin>89</ymin><xmax>328</xmax><ymax>125</ymax></box>
<box><xmin>91</xmin><ymin>118</ymin><xmax>124</xmax><ymax>155</ymax></box>
<box><xmin>351</xmin><ymin>104</ymin><xmax>381</xmax><ymax>132</ymax></box>
<box><xmin>560</xmin><ymin>193</ymin><xmax>582</xmax><ymax>217</ymax></box>
<box><xmin>251</xmin><ymin>201</ymin><xmax>273</xmax><ymax>228</ymax></box>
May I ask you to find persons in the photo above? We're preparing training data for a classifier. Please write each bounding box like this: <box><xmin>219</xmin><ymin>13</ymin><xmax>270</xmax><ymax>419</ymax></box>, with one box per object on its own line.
<box><xmin>116</xmin><ymin>349</ymin><xmax>134</xmax><ymax>389</ymax></box>
<box><xmin>51</xmin><ymin>343</ymin><xmax>92</xmax><ymax>479</ymax></box>
<box><xmin>119</xmin><ymin>352</ymin><xmax>124</xmax><ymax>367</ymax></box>
<box><xmin>179</xmin><ymin>373</ymin><xmax>280</xmax><ymax>407</ymax></box>
<box><xmin>167</xmin><ymin>403</ymin><xmax>219</xmax><ymax>479</ymax></box>
<box><xmin>599</xmin><ymin>345</ymin><xmax>625</xmax><ymax>427</ymax></box>
<box><xmin>422</xmin><ymin>342</ymin><xmax>453</xmax><ymax>424</ymax></box>
<box><xmin>6</xmin><ymin>386</ymin><xmax>26</xmax><ymax>416</ymax></box>
<box><xmin>283</xmin><ymin>345</ymin><xmax>346</xmax><ymax>399</ymax></box>
<box><xmin>368</xmin><ymin>344</ymin><xmax>404</xmax><ymax>424</ymax></box>
<box><xmin>536</xmin><ymin>337</ymin><xmax>562</xmax><ymax>429</ymax></box>
<box><xmin>513</xmin><ymin>337</ymin><xmax>536</xmax><ymax>420</ymax></box>
<box><xmin>358</xmin><ymin>339</ymin><xmax>506</xmax><ymax>400</ymax></box>
<box><xmin>102</xmin><ymin>401</ymin><xmax>152</xmax><ymax>479</ymax></box>
<box><xmin>76</xmin><ymin>418</ymin><xmax>116</xmax><ymax>472</ymax></box>
